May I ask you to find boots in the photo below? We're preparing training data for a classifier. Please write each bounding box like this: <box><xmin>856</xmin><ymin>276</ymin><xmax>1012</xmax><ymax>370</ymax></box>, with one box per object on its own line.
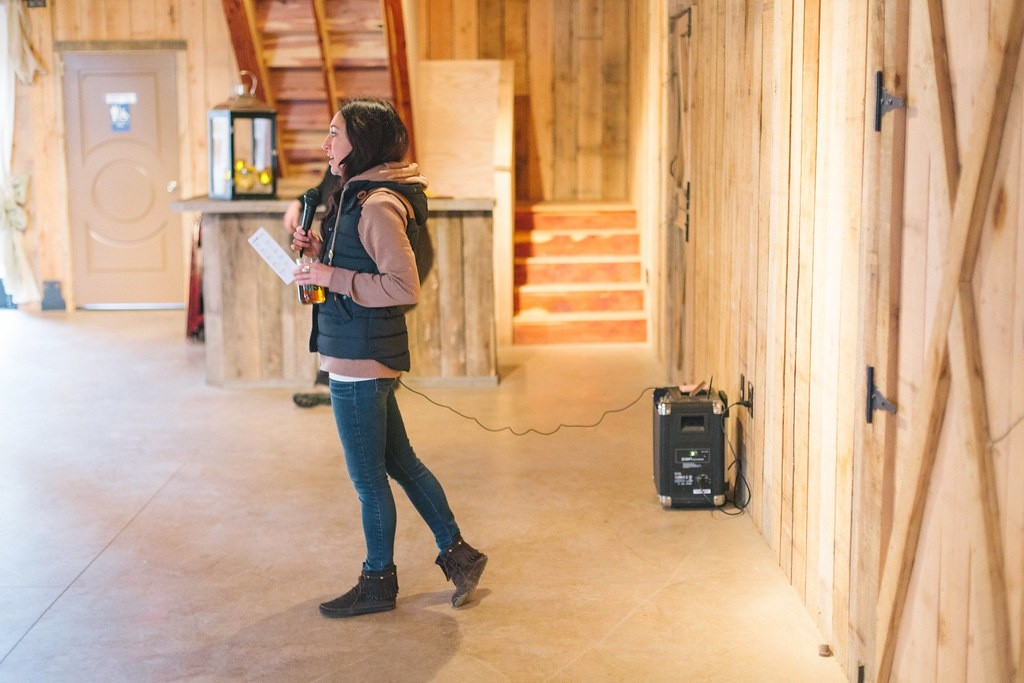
<box><xmin>434</xmin><ymin>535</ymin><xmax>488</xmax><ymax>606</ymax></box>
<box><xmin>319</xmin><ymin>562</ymin><xmax>399</xmax><ymax>618</ymax></box>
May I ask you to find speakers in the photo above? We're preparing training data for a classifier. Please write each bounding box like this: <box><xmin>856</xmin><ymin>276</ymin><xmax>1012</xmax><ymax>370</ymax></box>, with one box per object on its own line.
<box><xmin>652</xmin><ymin>385</ymin><xmax>727</xmax><ymax>509</ymax></box>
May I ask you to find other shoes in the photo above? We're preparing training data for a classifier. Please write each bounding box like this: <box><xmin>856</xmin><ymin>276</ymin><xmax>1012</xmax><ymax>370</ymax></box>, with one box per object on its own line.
<box><xmin>293</xmin><ymin>381</ymin><xmax>331</xmax><ymax>408</ymax></box>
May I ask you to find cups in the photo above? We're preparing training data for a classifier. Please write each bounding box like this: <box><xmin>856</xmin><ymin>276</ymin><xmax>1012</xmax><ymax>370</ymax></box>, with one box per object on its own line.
<box><xmin>296</xmin><ymin>255</ymin><xmax>326</xmax><ymax>304</ymax></box>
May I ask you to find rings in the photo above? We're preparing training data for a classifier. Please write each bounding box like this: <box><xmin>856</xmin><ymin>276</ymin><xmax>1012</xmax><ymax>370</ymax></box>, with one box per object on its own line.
<box><xmin>291</xmin><ymin>244</ymin><xmax>295</xmax><ymax>250</ymax></box>
<box><xmin>302</xmin><ymin>265</ymin><xmax>310</xmax><ymax>273</ymax></box>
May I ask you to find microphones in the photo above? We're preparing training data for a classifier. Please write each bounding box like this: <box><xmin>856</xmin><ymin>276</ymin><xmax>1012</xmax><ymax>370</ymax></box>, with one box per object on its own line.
<box><xmin>298</xmin><ymin>186</ymin><xmax>321</xmax><ymax>257</ymax></box>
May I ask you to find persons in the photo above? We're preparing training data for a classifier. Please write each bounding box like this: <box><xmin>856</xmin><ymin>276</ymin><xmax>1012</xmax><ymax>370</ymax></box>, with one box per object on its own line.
<box><xmin>284</xmin><ymin>102</ymin><xmax>488</xmax><ymax>618</ymax></box>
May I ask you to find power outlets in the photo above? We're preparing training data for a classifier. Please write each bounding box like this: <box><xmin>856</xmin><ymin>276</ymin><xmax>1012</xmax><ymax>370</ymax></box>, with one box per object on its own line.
<box><xmin>739</xmin><ymin>373</ymin><xmax>746</xmax><ymax>407</ymax></box>
<box><xmin>746</xmin><ymin>381</ymin><xmax>753</xmax><ymax>418</ymax></box>
